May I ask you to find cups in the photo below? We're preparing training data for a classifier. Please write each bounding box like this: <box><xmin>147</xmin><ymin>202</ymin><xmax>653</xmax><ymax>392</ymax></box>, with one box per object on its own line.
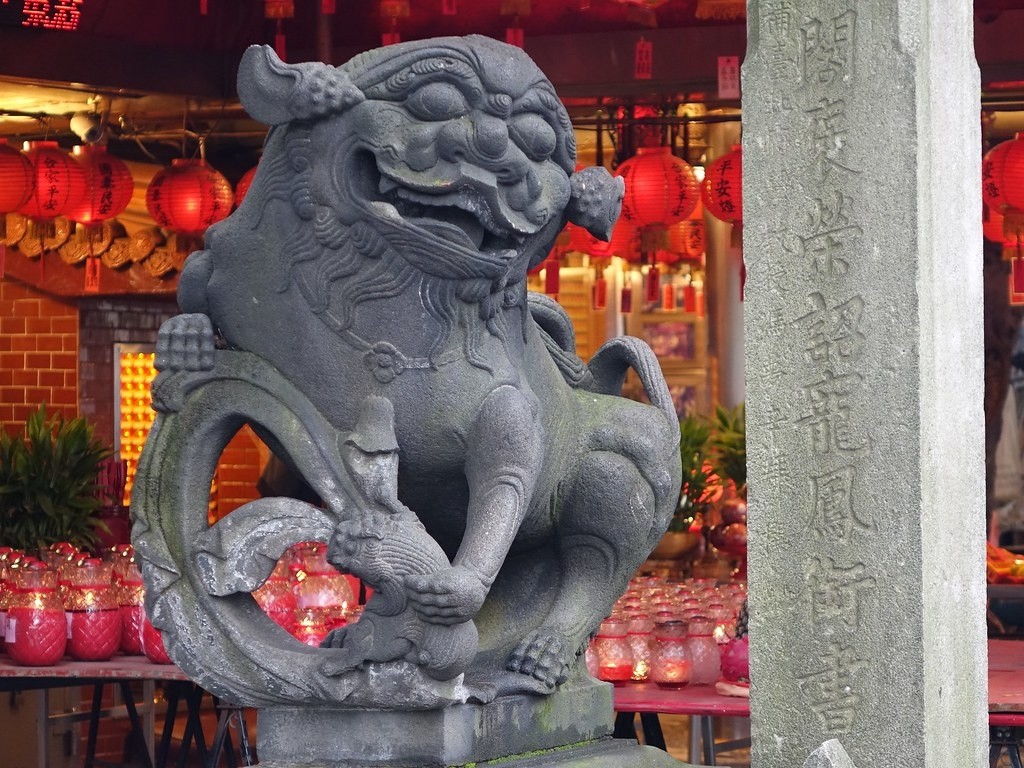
<box><xmin>95</xmin><ymin>462</ymin><xmax>127</xmax><ymax>516</ymax></box>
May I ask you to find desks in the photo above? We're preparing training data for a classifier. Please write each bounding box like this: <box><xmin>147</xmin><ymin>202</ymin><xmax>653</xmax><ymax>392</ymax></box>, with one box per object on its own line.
<box><xmin>0</xmin><ymin>652</ymin><xmax>752</xmax><ymax>768</ymax></box>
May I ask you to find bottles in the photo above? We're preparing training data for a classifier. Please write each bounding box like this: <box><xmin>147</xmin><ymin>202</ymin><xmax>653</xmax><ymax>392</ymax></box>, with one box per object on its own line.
<box><xmin>251</xmin><ymin>542</ymin><xmax>364</xmax><ymax>649</ymax></box>
<box><xmin>0</xmin><ymin>542</ymin><xmax>173</xmax><ymax>665</ymax></box>
<box><xmin>585</xmin><ymin>576</ymin><xmax>748</xmax><ymax>689</ymax></box>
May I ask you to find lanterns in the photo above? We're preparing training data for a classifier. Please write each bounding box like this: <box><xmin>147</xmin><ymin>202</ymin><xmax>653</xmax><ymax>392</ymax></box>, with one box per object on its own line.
<box><xmin>2</xmin><ymin>136</ymin><xmax>1024</xmax><ymax>314</ymax></box>
